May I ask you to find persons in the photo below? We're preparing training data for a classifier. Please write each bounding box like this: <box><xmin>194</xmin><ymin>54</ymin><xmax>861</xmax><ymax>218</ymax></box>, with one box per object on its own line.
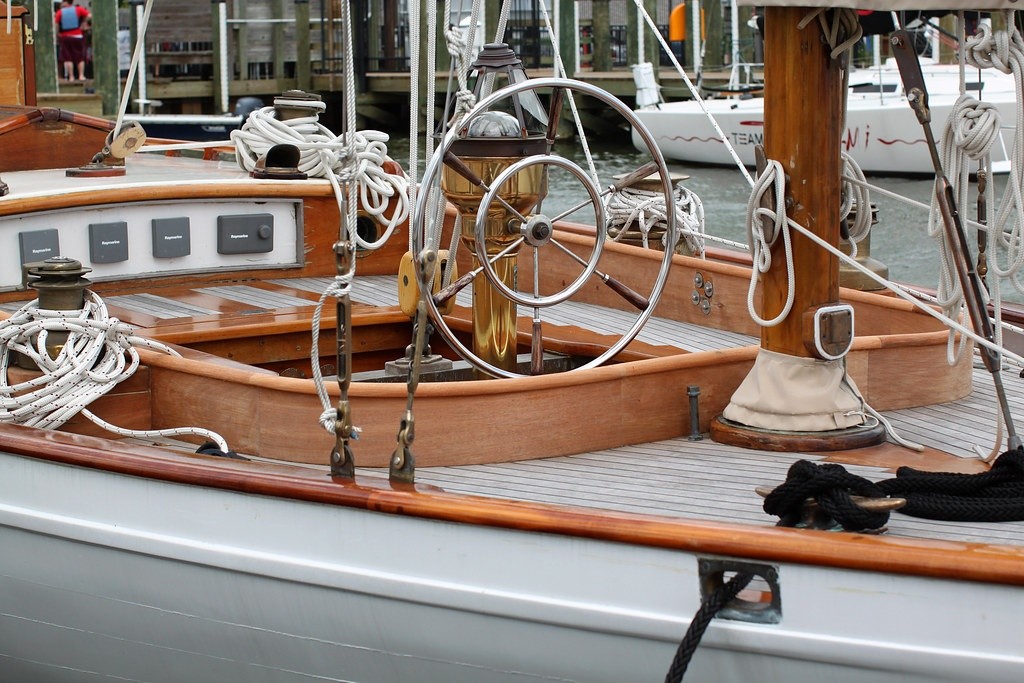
<box><xmin>54</xmin><ymin>0</ymin><xmax>92</xmax><ymax>81</ymax></box>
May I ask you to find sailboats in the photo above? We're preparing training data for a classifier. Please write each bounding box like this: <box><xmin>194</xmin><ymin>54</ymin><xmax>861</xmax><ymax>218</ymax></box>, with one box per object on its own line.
<box><xmin>0</xmin><ymin>0</ymin><xmax>1024</xmax><ymax>683</ymax></box>
<box><xmin>632</xmin><ymin>1</ymin><xmax>1024</xmax><ymax>182</ymax></box>
